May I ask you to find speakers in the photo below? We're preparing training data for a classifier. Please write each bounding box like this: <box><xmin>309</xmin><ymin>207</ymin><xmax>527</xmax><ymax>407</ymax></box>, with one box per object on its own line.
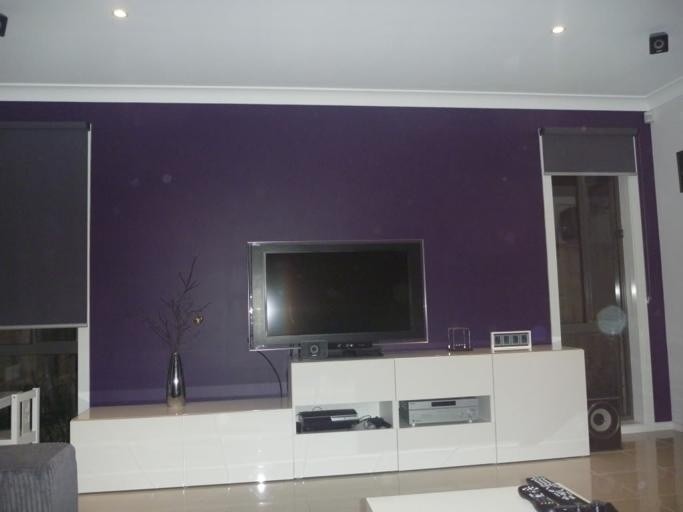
<box><xmin>0</xmin><ymin>14</ymin><xmax>8</xmax><ymax>36</ymax></box>
<box><xmin>587</xmin><ymin>390</ymin><xmax>622</xmax><ymax>451</ymax></box>
<box><xmin>650</xmin><ymin>34</ymin><xmax>668</xmax><ymax>54</ymax></box>
<box><xmin>303</xmin><ymin>340</ymin><xmax>328</xmax><ymax>360</ymax></box>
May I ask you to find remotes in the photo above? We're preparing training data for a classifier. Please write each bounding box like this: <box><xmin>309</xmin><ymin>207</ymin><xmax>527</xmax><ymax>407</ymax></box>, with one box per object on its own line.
<box><xmin>526</xmin><ymin>475</ymin><xmax>589</xmax><ymax>507</ymax></box>
<box><xmin>519</xmin><ymin>484</ymin><xmax>556</xmax><ymax>512</ymax></box>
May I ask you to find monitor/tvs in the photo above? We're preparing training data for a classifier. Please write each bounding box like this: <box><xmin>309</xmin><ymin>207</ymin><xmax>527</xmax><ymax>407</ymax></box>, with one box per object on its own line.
<box><xmin>247</xmin><ymin>238</ymin><xmax>429</xmax><ymax>358</ymax></box>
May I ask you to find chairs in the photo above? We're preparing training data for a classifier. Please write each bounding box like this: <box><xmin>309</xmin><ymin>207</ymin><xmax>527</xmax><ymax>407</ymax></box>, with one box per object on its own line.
<box><xmin>0</xmin><ymin>385</ymin><xmax>40</xmax><ymax>445</ymax></box>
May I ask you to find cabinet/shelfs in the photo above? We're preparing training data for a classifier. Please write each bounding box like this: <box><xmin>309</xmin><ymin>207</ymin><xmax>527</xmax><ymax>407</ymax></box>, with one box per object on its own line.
<box><xmin>69</xmin><ymin>397</ymin><xmax>291</xmax><ymax>494</ymax></box>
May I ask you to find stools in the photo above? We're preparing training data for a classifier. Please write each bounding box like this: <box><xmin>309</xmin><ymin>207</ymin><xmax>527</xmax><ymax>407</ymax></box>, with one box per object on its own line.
<box><xmin>0</xmin><ymin>443</ymin><xmax>79</xmax><ymax>512</ymax></box>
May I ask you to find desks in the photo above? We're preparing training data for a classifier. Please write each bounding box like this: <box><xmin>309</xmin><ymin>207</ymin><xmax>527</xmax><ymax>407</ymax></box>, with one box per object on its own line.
<box><xmin>0</xmin><ymin>389</ymin><xmax>23</xmax><ymax>409</ymax></box>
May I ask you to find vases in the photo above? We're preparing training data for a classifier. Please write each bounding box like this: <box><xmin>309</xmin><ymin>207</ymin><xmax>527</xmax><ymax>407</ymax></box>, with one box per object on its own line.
<box><xmin>165</xmin><ymin>351</ymin><xmax>186</xmax><ymax>408</ymax></box>
